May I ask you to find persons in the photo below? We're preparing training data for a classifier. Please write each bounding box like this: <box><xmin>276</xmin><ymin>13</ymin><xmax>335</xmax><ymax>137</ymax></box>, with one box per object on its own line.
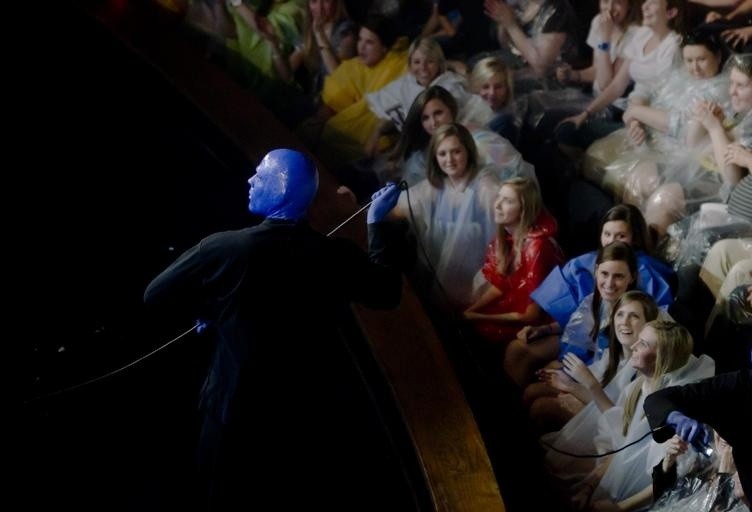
<box><xmin>165</xmin><ymin>3</ymin><xmax>751</xmax><ymax>510</ymax></box>
<box><xmin>140</xmin><ymin>148</ymin><xmax>421</xmax><ymax>510</ymax></box>
